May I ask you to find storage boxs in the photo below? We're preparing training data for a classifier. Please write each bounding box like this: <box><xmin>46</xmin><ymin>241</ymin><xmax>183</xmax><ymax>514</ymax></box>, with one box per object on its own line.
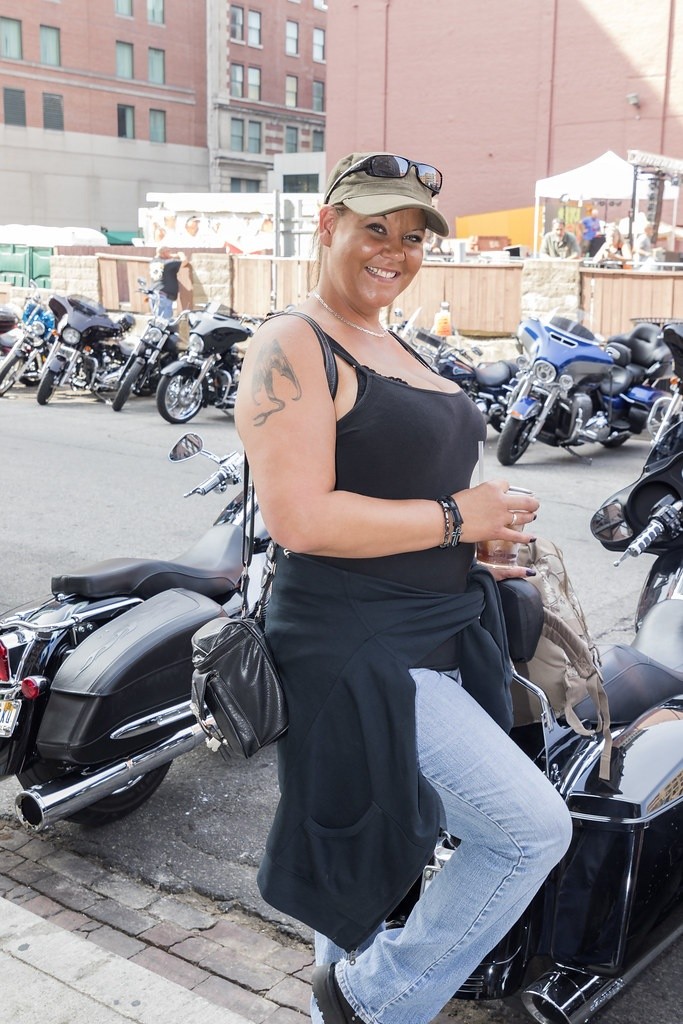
<box><xmin>36</xmin><ymin>588</ymin><xmax>228</xmax><ymax>766</ymax></box>
<box><xmin>537</xmin><ymin>693</ymin><xmax>683</xmax><ymax>984</ymax></box>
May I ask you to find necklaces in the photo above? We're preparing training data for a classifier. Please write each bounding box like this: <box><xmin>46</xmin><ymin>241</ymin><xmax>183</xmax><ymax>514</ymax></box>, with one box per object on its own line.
<box><xmin>310</xmin><ymin>289</ymin><xmax>387</xmax><ymax>339</ymax></box>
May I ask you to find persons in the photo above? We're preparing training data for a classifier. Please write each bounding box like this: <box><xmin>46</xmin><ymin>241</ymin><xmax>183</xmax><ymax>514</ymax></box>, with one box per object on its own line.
<box><xmin>149</xmin><ymin>246</ymin><xmax>189</xmax><ymax>319</ymax></box>
<box><xmin>579</xmin><ymin>208</ymin><xmax>655</xmax><ymax>269</ymax></box>
<box><xmin>235</xmin><ymin>150</ymin><xmax>574</xmax><ymax>1024</ymax></box>
<box><xmin>539</xmin><ymin>217</ymin><xmax>581</xmax><ymax>261</ymax></box>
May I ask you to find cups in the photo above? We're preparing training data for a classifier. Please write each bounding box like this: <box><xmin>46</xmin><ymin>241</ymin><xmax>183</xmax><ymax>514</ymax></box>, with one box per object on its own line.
<box><xmin>476</xmin><ymin>486</ymin><xmax>534</xmax><ymax>570</ymax></box>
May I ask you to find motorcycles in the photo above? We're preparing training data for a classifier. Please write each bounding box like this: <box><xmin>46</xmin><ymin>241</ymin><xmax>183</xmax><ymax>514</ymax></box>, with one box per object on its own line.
<box><xmin>496</xmin><ymin>306</ymin><xmax>677</xmax><ymax>466</ymax></box>
<box><xmin>0</xmin><ymin>430</ymin><xmax>277</xmax><ymax>828</ymax></box>
<box><xmin>386</xmin><ymin>422</ymin><xmax>683</xmax><ymax>1024</ymax></box>
<box><xmin>387</xmin><ymin>306</ymin><xmax>523</xmax><ymax>434</ymax></box>
<box><xmin>0</xmin><ymin>278</ymin><xmax>266</xmax><ymax>425</ymax></box>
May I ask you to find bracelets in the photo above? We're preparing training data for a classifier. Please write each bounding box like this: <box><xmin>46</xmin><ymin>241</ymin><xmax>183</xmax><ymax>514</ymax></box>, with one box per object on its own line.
<box><xmin>429</xmin><ymin>495</ymin><xmax>464</xmax><ymax>548</ymax></box>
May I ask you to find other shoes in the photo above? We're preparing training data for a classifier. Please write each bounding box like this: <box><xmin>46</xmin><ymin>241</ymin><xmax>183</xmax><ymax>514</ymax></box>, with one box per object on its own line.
<box><xmin>310</xmin><ymin>962</ymin><xmax>366</xmax><ymax>1024</ymax></box>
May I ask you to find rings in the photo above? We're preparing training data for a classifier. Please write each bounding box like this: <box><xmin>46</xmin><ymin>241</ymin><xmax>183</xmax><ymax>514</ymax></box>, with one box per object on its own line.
<box><xmin>509</xmin><ymin>511</ymin><xmax>517</xmax><ymax>525</ymax></box>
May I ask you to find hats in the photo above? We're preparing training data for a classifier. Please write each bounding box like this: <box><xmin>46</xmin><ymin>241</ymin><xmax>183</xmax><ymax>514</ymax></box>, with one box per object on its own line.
<box><xmin>324</xmin><ymin>152</ymin><xmax>449</xmax><ymax>237</ymax></box>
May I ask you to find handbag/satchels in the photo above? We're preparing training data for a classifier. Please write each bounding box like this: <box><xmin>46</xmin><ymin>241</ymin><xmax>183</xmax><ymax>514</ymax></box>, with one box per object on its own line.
<box><xmin>188</xmin><ymin>616</ymin><xmax>287</xmax><ymax>765</ymax></box>
<box><xmin>515</xmin><ymin>538</ymin><xmax>611</xmax><ymax>780</ymax></box>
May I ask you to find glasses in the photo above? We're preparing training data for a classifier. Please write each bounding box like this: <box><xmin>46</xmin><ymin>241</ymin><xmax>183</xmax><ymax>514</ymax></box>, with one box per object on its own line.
<box><xmin>324</xmin><ymin>154</ymin><xmax>442</xmax><ymax>205</ymax></box>
